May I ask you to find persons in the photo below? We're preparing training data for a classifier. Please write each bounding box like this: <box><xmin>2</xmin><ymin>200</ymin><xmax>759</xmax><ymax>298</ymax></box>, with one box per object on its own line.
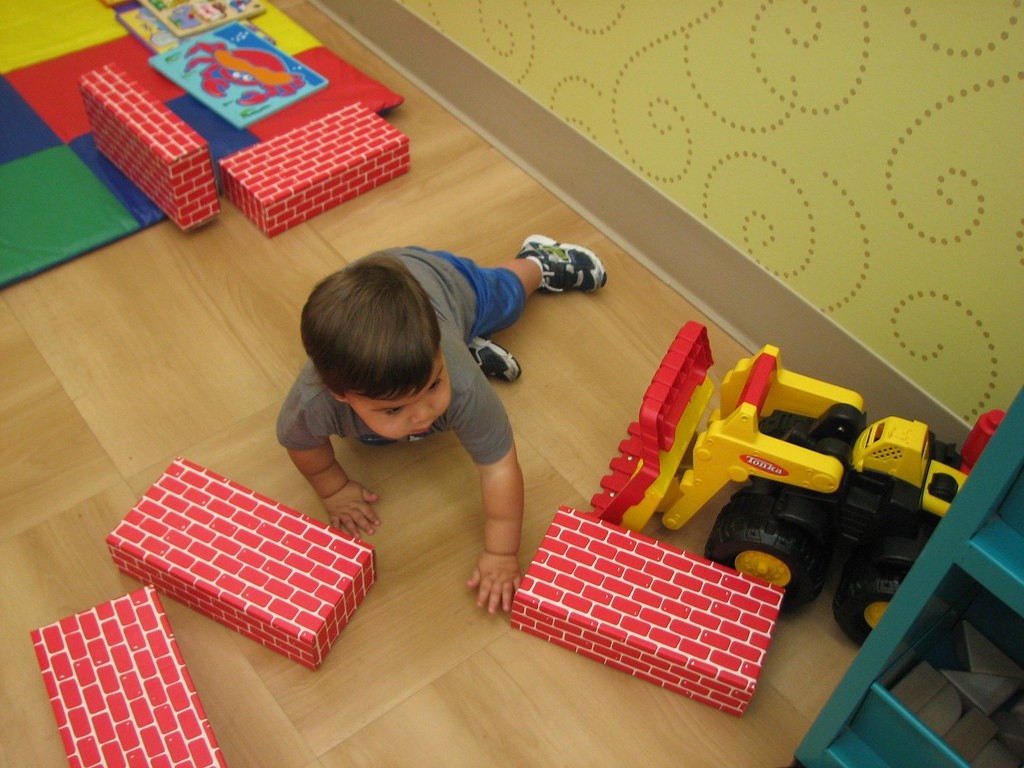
<box><xmin>277</xmin><ymin>233</ymin><xmax>606</xmax><ymax>614</ymax></box>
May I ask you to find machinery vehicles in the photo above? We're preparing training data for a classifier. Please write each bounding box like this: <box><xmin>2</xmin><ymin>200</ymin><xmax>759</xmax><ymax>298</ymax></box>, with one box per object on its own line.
<box><xmin>584</xmin><ymin>320</ymin><xmax>1005</xmax><ymax>647</ymax></box>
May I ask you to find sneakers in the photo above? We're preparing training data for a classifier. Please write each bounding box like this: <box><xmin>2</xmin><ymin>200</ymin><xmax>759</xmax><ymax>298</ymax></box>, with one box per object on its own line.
<box><xmin>467</xmin><ymin>335</ymin><xmax>521</xmax><ymax>383</ymax></box>
<box><xmin>515</xmin><ymin>234</ymin><xmax>607</xmax><ymax>293</ymax></box>
